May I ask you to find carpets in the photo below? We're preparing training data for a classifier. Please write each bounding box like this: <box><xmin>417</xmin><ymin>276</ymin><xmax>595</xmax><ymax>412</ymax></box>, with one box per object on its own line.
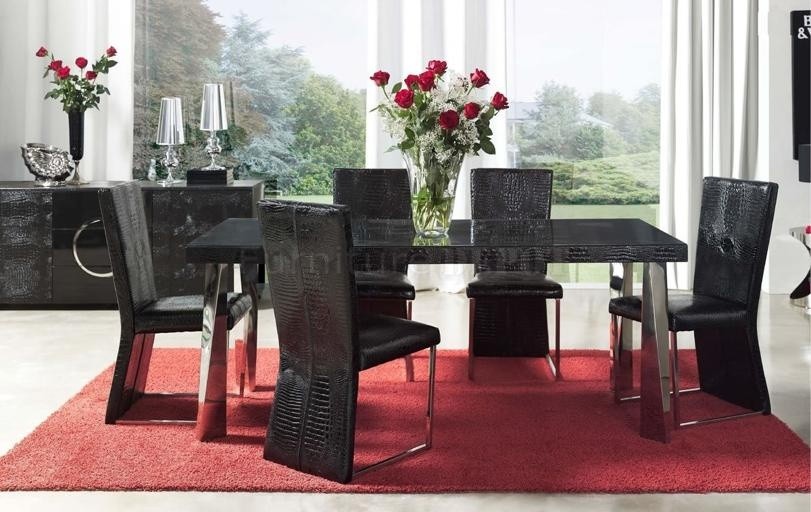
<box><xmin>0</xmin><ymin>348</ymin><xmax>811</xmax><ymax>494</ymax></box>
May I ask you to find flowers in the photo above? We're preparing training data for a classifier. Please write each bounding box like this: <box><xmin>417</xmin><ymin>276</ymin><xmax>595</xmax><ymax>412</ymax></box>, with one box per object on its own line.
<box><xmin>370</xmin><ymin>59</ymin><xmax>516</xmax><ymax>228</ymax></box>
<box><xmin>36</xmin><ymin>45</ymin><xmax>117</xmax><ymax>115</ymax></box>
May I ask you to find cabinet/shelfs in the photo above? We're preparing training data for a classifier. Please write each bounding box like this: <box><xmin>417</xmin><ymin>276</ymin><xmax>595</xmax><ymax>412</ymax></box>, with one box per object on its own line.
<box><xmin>0</xmin><ymin>180</ymin><xmax>265</xmax><ymax>310</ymax></box>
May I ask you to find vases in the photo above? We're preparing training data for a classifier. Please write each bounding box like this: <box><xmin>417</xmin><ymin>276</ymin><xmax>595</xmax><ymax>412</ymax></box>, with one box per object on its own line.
<box><xmin>67</xmin><ymin>112</ymin><xmax>88</xmax><ymax>185</ymax></box>
<box><xmin>412</xmin><ymin>233</ymin><xmax>452</xmax><ymax>250</ymax></box>
<box><xmin>400</xmin><ymin>149</ymin><xmax>460</xmax><ymax>238</ymax></box>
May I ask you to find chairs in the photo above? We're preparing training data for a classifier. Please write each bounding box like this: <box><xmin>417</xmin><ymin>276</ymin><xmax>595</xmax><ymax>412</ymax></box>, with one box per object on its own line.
<box><xmin>332</xmin><ymin>167</ymin><xmax>416</xmax><ymax>321</ymax></box>
<box><xmin>465</xmin><ymin>167</ymin><xmax>564</xmax><ymax>384</ymax></box>
<box><xmin>605</xmin><ymin>175</ymin><xmax>780</xmax><ymax>433</ymax></box>
<box><xmin>255</xmin><ymin>198</ymin><xmax>441</xmax><ymax>484</ymax></box>
<box><xmin>98</xmin><ymin>179</ymin><xmax>253</xmax><ymax>425</ymax></box>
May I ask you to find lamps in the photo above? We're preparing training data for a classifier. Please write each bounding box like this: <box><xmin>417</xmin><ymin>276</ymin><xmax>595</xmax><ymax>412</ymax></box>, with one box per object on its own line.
<box><xmin>187</xmin><ymin>83</ymin><xmax>235</xmax><ymax>183</ymax></box>
<box><xmin>155</xmin><ymin>96</ymin><xmax>185</xmax><ymax>179</ymax></box>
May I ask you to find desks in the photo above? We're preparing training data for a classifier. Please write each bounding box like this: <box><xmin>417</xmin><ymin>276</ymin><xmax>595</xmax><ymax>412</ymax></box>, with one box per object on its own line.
<box><xmin>789</xmin><ymin>227</ymin><xmax>811</xmax><ymax>315</ymax></box>
<box><xmin>184</xmin><ymin>217</ymin><xmax>689</xmax><ymax>441</ymax></box>
